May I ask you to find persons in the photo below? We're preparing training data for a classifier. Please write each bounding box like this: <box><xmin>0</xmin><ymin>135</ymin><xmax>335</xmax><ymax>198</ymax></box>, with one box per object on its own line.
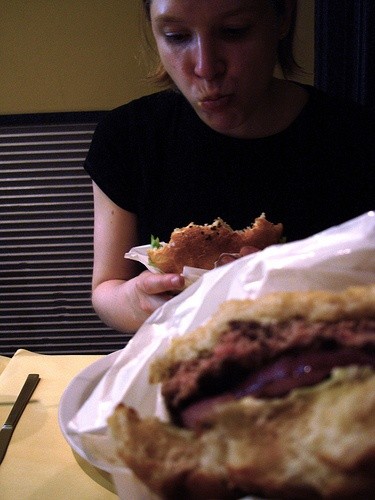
<box><xmin>82</xmin><ymin>0</ymin><xmax>375</xmax><ymax>335</ymax></box>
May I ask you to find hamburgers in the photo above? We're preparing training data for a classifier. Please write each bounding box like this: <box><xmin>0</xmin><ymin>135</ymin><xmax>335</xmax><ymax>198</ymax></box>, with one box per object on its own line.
<box><xmin>110</xmin><ymin>283</ymin><xmax>374</xmax><ymax>500</ymax></box>
<box><xmin>123</xmin><ymin>213</ymin><xmax>284</xmax><ymax>276</ymax></box>
